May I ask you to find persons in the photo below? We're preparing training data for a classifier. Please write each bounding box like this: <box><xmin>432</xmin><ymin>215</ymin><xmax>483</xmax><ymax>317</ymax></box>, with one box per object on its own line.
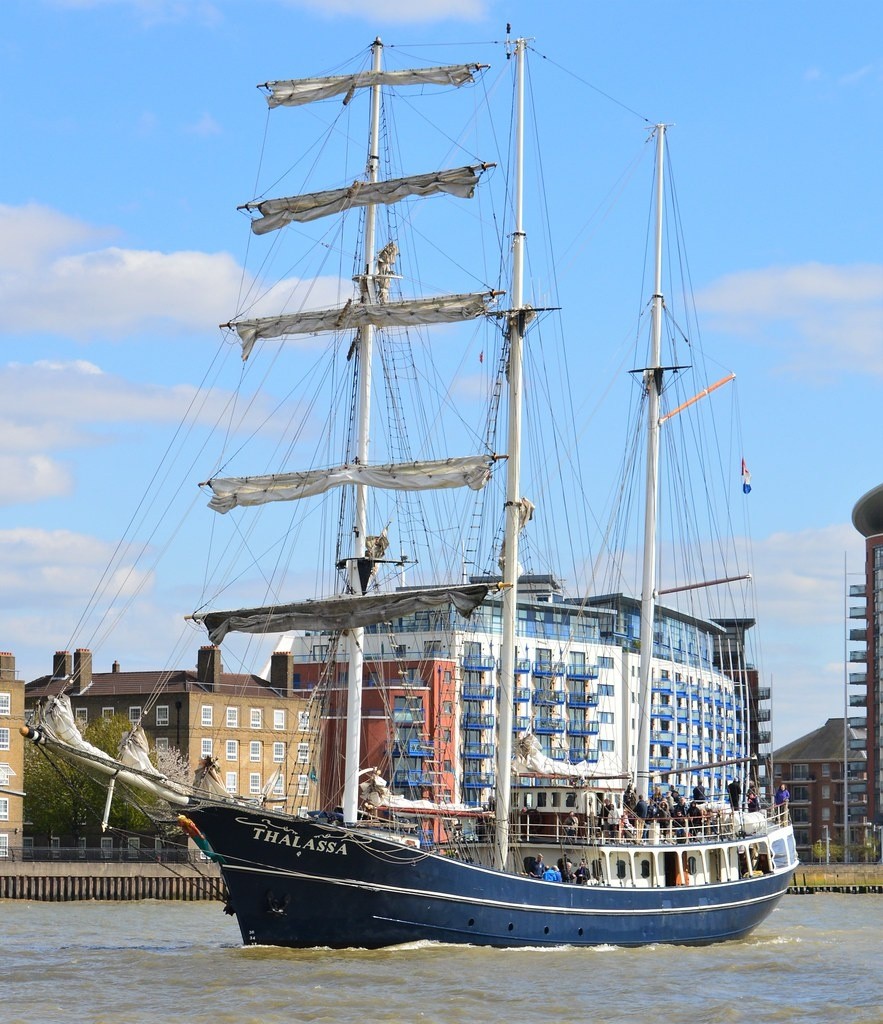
<box><xmin>598</xmin><ymin>779</ymin><xmax>705</xmax><ymax>844</ymax></box>
<box><xmin>728</xmin><ymin>777</ymin><xmax>761</xmax><ymax>835</ymax></box>
<box><xmin>775</xmin><ymin>784</ymin><xmax>790</xmax><ymax>822</ymax></box>
<box><xmin>529</xmin><ymin>853</ymin><xmax>591</xmax><ymax>885</ymax></box>
<box><xmin>562</xmin><ymin>811</ymin><xmax>579</xmax><ymax>840</ymax></box>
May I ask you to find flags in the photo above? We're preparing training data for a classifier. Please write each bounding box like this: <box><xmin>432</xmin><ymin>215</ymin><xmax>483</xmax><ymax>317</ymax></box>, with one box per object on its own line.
<box><xmin>741</xmin><ymin>458</ymin><xmax>751</xmax><ymax>495</ymax></box>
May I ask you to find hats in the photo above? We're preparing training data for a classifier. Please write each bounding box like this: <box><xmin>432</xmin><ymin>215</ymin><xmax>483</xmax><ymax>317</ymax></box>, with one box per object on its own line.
<box><xmin>734</xmin><ymin>777</ymin><xmax>738</xmax><ymax>781</ymax></box>
<box><xmin>537</xmin><ymin>854</ymin><xmax>543</xmax><ymax>857</ymax></box>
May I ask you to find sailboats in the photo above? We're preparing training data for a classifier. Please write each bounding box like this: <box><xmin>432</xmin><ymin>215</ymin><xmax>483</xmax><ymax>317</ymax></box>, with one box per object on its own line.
<box><xmin>20</xmin><ymin>33</ymin><xmax>813</xmax><ymax>961</ymax></box>
